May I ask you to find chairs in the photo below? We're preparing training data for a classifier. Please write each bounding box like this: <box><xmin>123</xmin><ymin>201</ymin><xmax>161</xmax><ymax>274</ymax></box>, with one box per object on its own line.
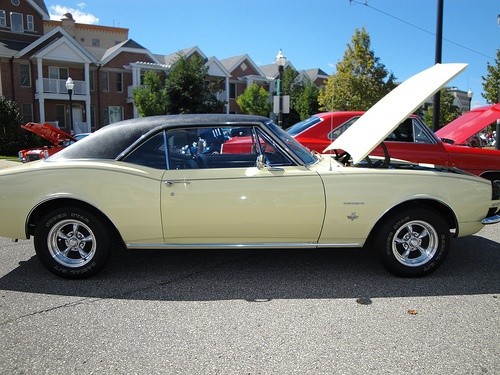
<box><xmin>187</xmin><ymin>155</ymin><xmax>207</xmax><ymax>168</ymax></box>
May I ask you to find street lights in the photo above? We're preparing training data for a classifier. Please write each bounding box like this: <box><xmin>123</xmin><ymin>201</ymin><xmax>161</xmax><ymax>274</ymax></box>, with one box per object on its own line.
<box><xmin>275</xmin><ymin>48</ymin><xmax>286</xmax><ymax>129</ymax></box>
<box><xmin>65</xmin><ymin>77</ymin><xmax>74</xmax><ymax>137</ymax></box>
<box><xmin>467</xmin><ymin>90</ymin><xmax>473</xmax><ymax>111</ymax></box>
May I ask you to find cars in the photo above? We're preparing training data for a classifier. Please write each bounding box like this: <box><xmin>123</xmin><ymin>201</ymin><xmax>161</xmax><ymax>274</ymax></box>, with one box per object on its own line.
<box><xmin>0</xmin><ymin>62</ymin><xmax>500</xmax><ymax>280</ymax></box>
<box><xmin>218</xmin><ymin>104</ymin><xmax>500</xmax><ymax>218</ymax></box>
<box><xmin>18</xmin><ymin>122</ymin><xmax>94</xmax><ymax>165</ymax></box>
